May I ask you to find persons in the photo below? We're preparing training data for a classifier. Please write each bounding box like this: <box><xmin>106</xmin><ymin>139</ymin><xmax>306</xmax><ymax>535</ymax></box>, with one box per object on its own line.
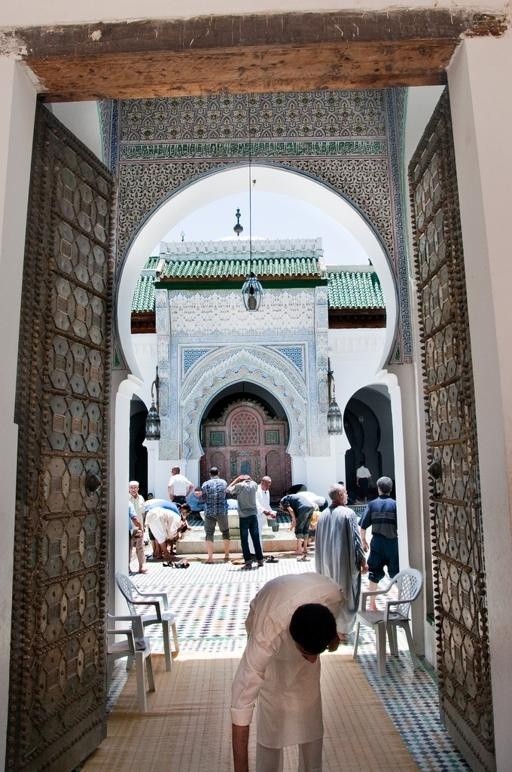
<box><xmin>354</xmin><ymin>462</ymin><xmax>373</xmax><ymax>501</ymax></box>
<box><xmin>226</xmin><ymin>571</ymin><xmax>349</xmax><ymax>772</ymax></box>
<box><xmin>357</xmin><ymin>471</ymin><xmax>400</xmax><ymax>612</ymax></box>
<box><xmin>310</xmin><ymin>483</ymin><xmax>369</xmax><ymax>648</ymax></box>
<box><xmin>126</xmin><ymin>465</ymin><xmax>357</xmax><ymax>577</ymax></box>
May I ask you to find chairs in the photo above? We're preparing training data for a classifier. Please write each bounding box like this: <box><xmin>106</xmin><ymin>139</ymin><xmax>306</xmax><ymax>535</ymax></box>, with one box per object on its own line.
<box><xmin>116</xmin><ymin>572</ymin><xmax>180</xmax><ymax>672</ymax></box>
<box><xmin>108</xmin><ymin>613</ymin><xmax>156</xmax><ymax>714</ymax></box>
<box><xmin>352</xmin><ymin>568</ymin><xmax>423</xmax><ymax>677</ymax></box>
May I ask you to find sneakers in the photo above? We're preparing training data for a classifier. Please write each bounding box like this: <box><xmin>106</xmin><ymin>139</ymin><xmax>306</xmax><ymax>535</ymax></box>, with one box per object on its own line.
<box><xmin>242</xmin><ymin>561</ymin><xmax>262</xmax><ymax>568</ymax></box>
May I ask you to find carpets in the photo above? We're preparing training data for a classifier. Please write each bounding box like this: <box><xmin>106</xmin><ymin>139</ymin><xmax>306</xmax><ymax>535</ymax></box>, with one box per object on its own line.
<box><xmin>81</xmin><ymin>652</ymin><xmax>419</xmax><ymax>772</ymax></box>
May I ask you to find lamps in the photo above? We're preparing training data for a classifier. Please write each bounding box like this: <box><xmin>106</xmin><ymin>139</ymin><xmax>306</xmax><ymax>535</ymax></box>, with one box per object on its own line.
<box><xmin>325</xmin><ymin>357</ymin><xmax>343</xmax><ymax>435</ymax></box>
<box><xmin>145</xmin><ymin>366</ymin><xmax>160</xmax><ymax>441</ymax></box>
<box><xmin>241</xmin><ymin>94</ymin><xmax>263</xmax><ymax>312</ymax></box>
